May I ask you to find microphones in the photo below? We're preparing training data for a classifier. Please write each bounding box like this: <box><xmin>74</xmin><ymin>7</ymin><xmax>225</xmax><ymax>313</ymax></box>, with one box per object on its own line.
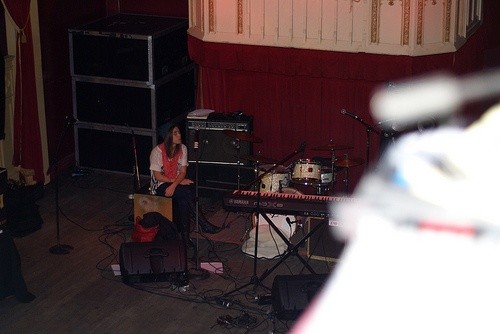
<box><xmin>294</xmin><ymin>144</ymin><xmax>305</xmax><ymax>153</ymax></box>
<box><xmin>341</xmin><ymin>109</ymin><xmax>358</xmax><ymax>119</ymax></box>
<box><xmin>193</xmin><ymin>127</ymin><xmax>200</xmax><ymax>152</ymax></box>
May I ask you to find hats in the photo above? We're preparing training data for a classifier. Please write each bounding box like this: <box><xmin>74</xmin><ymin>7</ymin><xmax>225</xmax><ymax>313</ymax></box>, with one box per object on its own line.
<box><xmin>159</xmin><ymin>122</ymin><xmax>176</xmax><ymax>140</ymax></box>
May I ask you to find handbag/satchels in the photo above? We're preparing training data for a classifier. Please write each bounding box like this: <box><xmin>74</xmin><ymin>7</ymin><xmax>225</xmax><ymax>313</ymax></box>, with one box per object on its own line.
<box><xmin>142</xmin><ymin>212</ymin><xmax>178</xmax><ymax>256</ymax></box>
<box><xmin>131</xmin><ymin>216</ymin><xmax>161</xmax><ymax>241</ymax></box>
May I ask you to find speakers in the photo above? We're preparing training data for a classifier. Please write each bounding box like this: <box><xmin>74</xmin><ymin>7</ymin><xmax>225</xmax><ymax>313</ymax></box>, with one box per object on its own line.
<box><xmin>134</xmin><ymin>191</ymin><xmax>180</xmax><ymax>226</ymax></box>
<box><xmin>184</xmin><ymin>112</ymin><xmax>254</xmax><ymax>165</ymax></box>
<box><xmin>271</xmin><ymin>274</ymin><xmax>329</xmax><ymax>320</ymax></box>
<box><xmin>118</xmin><ymin>240</ymin><xmax>187</xmax><ymax>284</ymax></box>
<box><xmin>307</xmin><ymin>217</ymin><xmax>348</xmax><ymax>262</ymax></box>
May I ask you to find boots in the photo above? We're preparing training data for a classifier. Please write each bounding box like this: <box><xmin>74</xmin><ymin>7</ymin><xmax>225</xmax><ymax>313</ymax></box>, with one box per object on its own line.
<box><xmin>181</xmin><ymin>224</ymin><xmax>192</xmax><ymax>247</ymax></box>
<box><xmin>190</xmin><ymin>207</ymin><xmax>221</xmax><ymax>234</ymax></box>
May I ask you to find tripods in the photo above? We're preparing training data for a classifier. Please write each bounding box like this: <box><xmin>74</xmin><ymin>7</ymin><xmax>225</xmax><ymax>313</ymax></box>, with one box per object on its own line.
<box><xmin>224</xmin><ymin>148</ymin><xmax>300</xmax><ymax>297</ymax></box>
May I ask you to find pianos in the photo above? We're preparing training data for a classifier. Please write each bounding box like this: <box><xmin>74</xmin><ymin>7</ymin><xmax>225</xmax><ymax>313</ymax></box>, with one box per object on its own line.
<box><xmin>220</xmin><ymin>186</ymin><xmax>362</xmax><ymax>295</ymax></box>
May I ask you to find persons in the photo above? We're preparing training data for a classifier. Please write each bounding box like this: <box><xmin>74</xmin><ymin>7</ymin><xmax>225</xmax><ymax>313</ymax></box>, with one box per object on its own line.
<box><xmin>0</xmin><ymin>236</ymin><xmax>37</xmax><ymax>301</ymax></box>
<box><xmin>150</xmin><ymin>125</ymin><xmax>221</xmax><ymax>247</ymax></box>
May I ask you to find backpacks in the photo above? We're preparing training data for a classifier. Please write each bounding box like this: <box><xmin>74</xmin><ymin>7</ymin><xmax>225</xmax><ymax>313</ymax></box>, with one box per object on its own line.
<box><xmin>0</xmin><ymin>233</ymin><xmax>37</xmax><ymax>303</ymax></box>
<box><xmin>6</xmin><ymin>196</ymin><xmax>44</xmax><ymax>232</ymax></box>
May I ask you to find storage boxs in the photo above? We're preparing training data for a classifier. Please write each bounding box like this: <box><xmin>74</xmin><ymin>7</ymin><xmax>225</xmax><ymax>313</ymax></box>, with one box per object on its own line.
<box><xmin>72</xmin><ymin>64</ymin><xmax>197</xmax><ymax>131</ymax></box>
<box><xmin>74</xmin><ymin>109</ymin><xmax>194</xmax><ymax>176</ymax></box>
<box><xmin>67</xmin><ymin>12</ymin><xmax>190</xmax><ymax>85</ymax></box>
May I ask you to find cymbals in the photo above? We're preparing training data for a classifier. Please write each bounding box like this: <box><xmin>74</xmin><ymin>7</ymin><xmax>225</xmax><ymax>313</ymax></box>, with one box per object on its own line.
<box><xmin>309</xmin><ymin>144</ymin><xmax>355</xmax><ymax>151</ymax></box>
<box><xmin>333</xmin><ymin>157</ymin><xmax>366</xmax><ymax>168</ymax></box>
<box><xmin>237</xmin><ymin>153</ymin><xmax>279</xmax><ymax>164</ymax></box>
<box><xmin>223</xmin><ymin>128</ymin><xmax>264</xmax><ymax>144</ymax></box>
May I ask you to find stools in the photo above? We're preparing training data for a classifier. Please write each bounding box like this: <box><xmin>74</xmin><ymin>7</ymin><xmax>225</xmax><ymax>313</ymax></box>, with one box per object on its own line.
<box><xmin>133</xmin><ymin>194</ymin><xmax>173</xmax><ymax>223</ymax></box>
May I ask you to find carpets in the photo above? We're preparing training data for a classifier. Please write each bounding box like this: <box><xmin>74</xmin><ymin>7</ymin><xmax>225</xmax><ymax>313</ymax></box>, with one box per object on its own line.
<box><xmin>190</xmin><ymin>209</ymin><xmax>307</xmax><ymax>256</ymax></box>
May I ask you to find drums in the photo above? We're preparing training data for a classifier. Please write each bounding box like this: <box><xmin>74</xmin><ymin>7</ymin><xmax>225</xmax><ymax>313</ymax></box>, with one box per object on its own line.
<box><xmin>253</xmin><ymin>164</ymin><xmax>290</xmax><ymax>194</ymax></box>
<box><xmin>290</xmin><ymin>158</ymin><xmax>322</xmax><ymax>185</ymax></box>
<box><xmin>249</xmin><ymin>188</ymin><xmax>305</xmax><ymax>239</ymax></box>
<box><xmin>320</xmin><ymin>165</ymin><xmax>338</xmax><ymax>187</ymax></box>
